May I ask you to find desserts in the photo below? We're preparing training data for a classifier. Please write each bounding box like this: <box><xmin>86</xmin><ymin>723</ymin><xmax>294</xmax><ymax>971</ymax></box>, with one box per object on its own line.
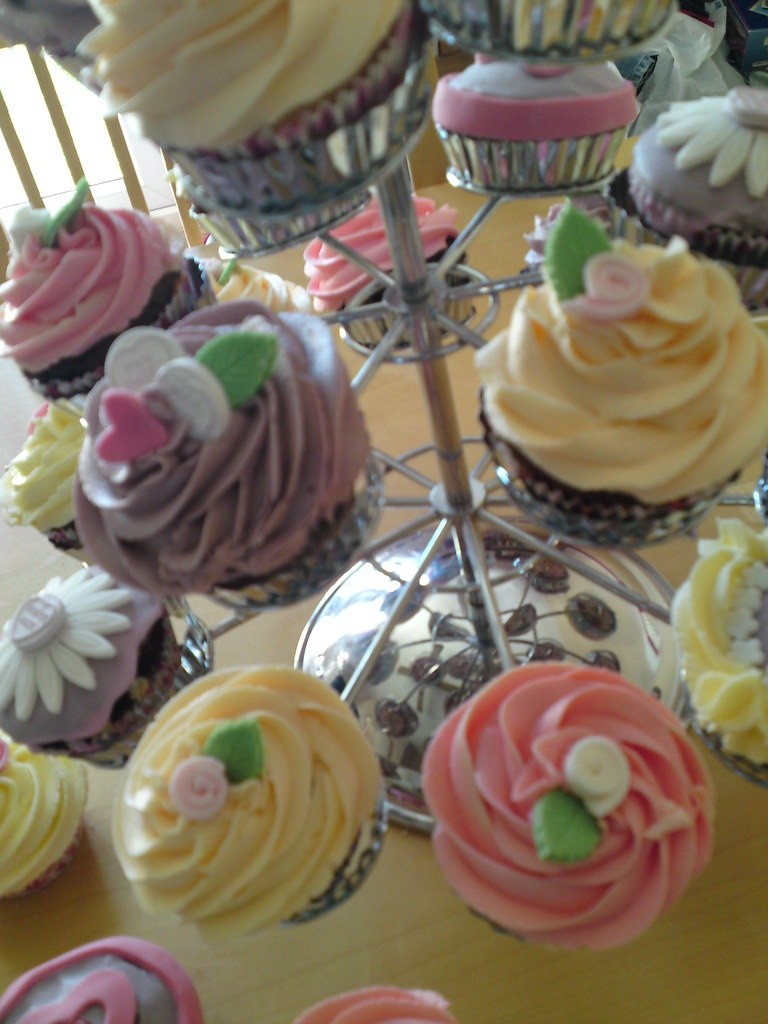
<box><xmin>0</xmin><ymin>0</ymin><xmax>768</xmax><ymax>1024</ymax></box>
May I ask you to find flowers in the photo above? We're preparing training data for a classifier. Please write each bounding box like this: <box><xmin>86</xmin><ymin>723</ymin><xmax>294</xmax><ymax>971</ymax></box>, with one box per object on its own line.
<box><xmin>1</xmin><ymin>0</ymin><xmax>767</xmax><ymax>1024</ymax></box>
